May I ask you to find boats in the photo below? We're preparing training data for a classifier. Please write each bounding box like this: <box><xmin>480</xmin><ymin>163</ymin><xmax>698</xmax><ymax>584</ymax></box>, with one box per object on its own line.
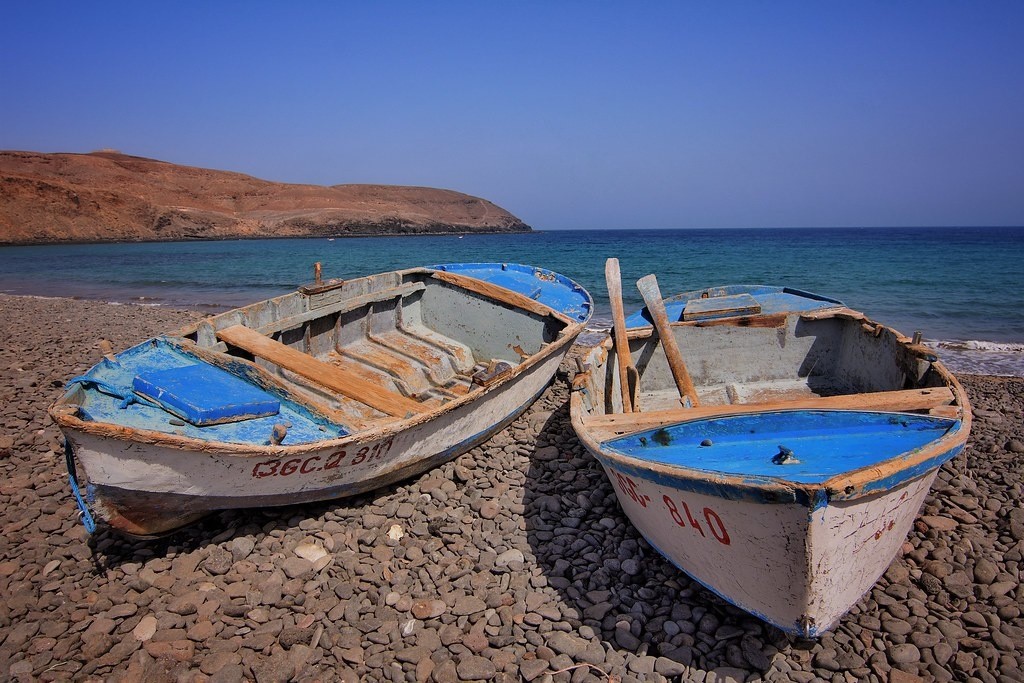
<box><xmin>569</xmin><ymin>282</ymin><xmax>976</xmax><ymax>644</ymax></box>
<box><xmin>45</xmin><ymin>262</ymin><xmax>595</xmax><ymax>576</ymax></box>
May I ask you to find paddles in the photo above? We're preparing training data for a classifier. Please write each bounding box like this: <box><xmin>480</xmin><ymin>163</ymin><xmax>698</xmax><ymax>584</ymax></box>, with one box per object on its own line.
<box><xmin>636</xmin><ymin>272</ymin><xmax>699</xmax><ymax>408</ymax></box>
<box><xmin>604</xmin><ymin>258</ymin><xmax>641</xmax><ymax>414</ymax></box>
